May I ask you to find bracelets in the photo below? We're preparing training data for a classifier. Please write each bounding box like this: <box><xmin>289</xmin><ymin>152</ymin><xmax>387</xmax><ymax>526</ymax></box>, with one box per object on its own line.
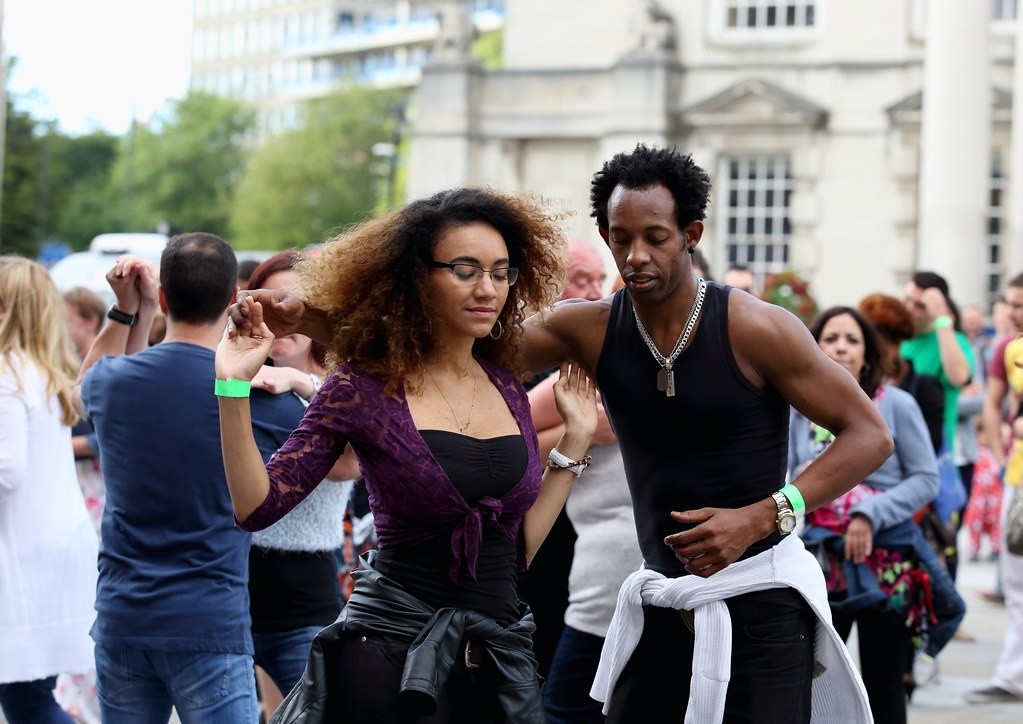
<box><xmin>214</xmin><ymin>376</ymin><xmax>252</xmax><ymax>399</ymax></box>
<box><xmin>544</xmin><ymin>456</ymin><xmax>593</xmax><ymax>471</ymax></box>
<box><xmin>930</xmin><ymin>315</ymin><xmax>954</xmax><ymax>330</ymax></box>
<box><xmin>105</xmin><ymin>305</ymin><xmax>138</xmax><ymax>328</ymax></box>
<box><xmin>779</xmin><ymin>483</ymin><xmax>806</xmax><ymax>522</ymax></box>
<box><xmin>304</xmin><ymin>369</ymin><xmax>323</xmax><ymax>404</ymax></box>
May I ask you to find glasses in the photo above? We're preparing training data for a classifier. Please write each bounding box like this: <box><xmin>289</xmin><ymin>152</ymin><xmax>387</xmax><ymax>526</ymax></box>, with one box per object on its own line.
<box><xmin>427</xmin><ymin>258</ymin><xmax>521</xmax><ymax>287</ymax></box>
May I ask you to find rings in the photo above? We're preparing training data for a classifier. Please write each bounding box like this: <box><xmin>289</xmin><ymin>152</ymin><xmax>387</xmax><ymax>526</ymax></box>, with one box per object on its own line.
<box><xmin>250</xmin><ymin>334</ymin><xmax>264</xmax><ymax>340</ymax></box>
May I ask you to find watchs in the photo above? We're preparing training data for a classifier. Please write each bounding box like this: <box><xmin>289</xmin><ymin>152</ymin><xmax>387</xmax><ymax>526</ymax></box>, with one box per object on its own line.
<box><xmin>772</xmin><ymin>491</ymin><xmax>797</xmax><ymax>537</ymax></box>
<box><xmin>549</xmin><ymin>447</ymin><xmax>588</xmax><ymax>478</ymax></box>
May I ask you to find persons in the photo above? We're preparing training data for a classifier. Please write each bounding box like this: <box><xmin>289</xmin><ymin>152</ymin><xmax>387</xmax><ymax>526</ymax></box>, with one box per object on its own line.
<box><xmin>957</xmin><ymin>273</ymin><xmax>1023</xmax><ymax>701</ymax></box>
<box><xmin>896</xmin><ymin>272</ymin><xmax>975</xmax><ymax>583</ymax></box>
<box><xmin>784</xmin><ymin>307</ymin><xmax>966</xmax><ymax>722</ymax></box>
<box><xmin>70</xmin><ymin>232</ymin><xmax>363</xmax><ymax>724</ymax></box>
<box><xmin>226</xmin><ymin>139</ymin><xmax>897</xmax><ymax>723</ymax></box>
<box><xmin>58</xmin><ymin>282</ymin><xmax>106</xmax><ymax>528</ymax></box>
<box><xmin>539</xmin><ymin>230</ymin><xmax>759</xmax><ymax>312</ymax></box>
<box><xmin>211</xmin><ymin>190</ymin><xmax>598</xmax><ymax>724</ymax></box>
<box><xmin>235</xmin><ymin>259</ymin><xmax>260</xmax><ymax>294</ymax></box>
<box><xmin>859</xmin><ymin>293</ymin><xmax>942</xmax><ymax>478</ymax></box>
<box><xmin>0</xmin><ymin>251</ymin><xmax>103</xmax><ymax>723</ymax></box>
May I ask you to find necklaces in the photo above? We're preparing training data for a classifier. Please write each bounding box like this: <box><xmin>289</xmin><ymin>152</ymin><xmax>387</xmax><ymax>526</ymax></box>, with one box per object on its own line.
<box><xmin>631</xmin><ymin>277</ymin><xmax>707</xmax><ymax>397</ymax></box>
<box><xmin>419</xmin><ymin>359</ymin><xmax>477</xmax><ymax>433</ymax></box>
